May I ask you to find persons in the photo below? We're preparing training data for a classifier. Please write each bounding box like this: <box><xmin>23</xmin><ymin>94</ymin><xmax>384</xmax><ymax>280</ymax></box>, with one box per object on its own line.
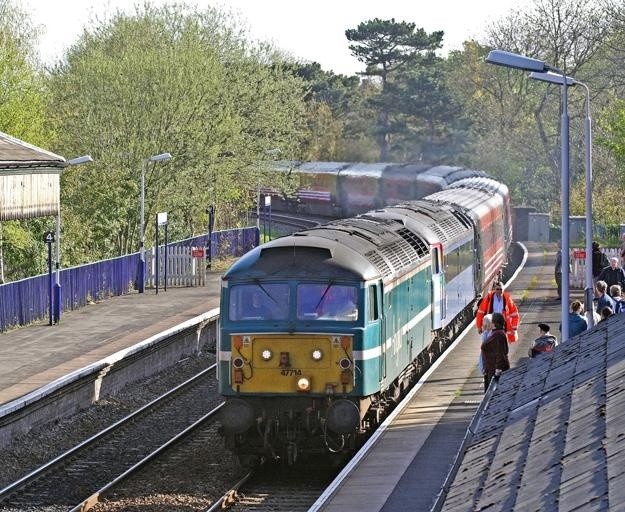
<box><xmin>322</xmin><ymin>286</ymin><xmax>355</xmax><ymax>316</ymax></box>
<box><xmin>478</xmin><ymin>314</ymin><xmax>496</xmax><ymax>392</ymax></box>
<box><xmin>242</xmin><ymin>290</ymin><xmax>269</xmax><ymax>318</ymax></box>
<box><xmin>476</xmin><ymin>281</ymin><xmax>520</xmax><ymax>344</ymax></box>
<box><xmin>554</xmin><ymin>241</ymin><xmax>625</xmax><ymax>344</ymax></box>
<box><xmin>528</xmin><ymin>324</ymin><xmax>558</xmax><ymax>358</ymax></box>
<box><xmin>481</xmin><ymin>313</ymin><xmax>510</xmax><ymax>385</ymax></box>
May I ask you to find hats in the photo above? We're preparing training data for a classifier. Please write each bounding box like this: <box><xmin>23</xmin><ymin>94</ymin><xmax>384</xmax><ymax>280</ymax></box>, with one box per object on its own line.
<box><xmin>537</xmin><ymin>323</ymin><xmax>551</xmax><ymax>333</ymax></box>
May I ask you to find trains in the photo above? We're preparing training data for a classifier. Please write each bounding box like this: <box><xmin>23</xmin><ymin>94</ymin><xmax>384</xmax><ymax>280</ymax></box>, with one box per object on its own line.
<box><xmin>211</xmin><ymin>152</ymin><xmax>516</xmax><ymax>476</ymax></box>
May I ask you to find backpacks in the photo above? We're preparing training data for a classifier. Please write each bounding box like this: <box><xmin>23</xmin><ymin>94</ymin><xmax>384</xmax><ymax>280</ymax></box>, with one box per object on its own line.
<box><xmin>612</xmin><ymin>296</ymin><xmax>625</xmax><ymax>314</ymax></box>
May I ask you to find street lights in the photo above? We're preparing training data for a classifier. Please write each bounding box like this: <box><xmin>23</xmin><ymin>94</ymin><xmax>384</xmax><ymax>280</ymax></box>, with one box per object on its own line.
<box><xmin>48</xmin><ymin>152</ymin><xmax>93</xmax><ymax>325</ymax></box>
<box><xmin>480</xmin><ymin>48</ymin><xmax>574</xmax><ymax>349</ymax></box>
<box><xmin>255</xmin><ymin>147</ymin><xmax>281</xmax><ymax>246</ymax></box>
<box><xmin>525</xmin><ymin>62</ymin><xmax>598</xmax><ymax>334</ymax></box>
<box><xmin>206</xmin><ymin>150</ymin><xmax>234</xmax><ymax>270</ymax></box>
<box><xmin>135</xmin><ymin>152</ymin><xmax>173</xmax><ymax>293</ymax></box>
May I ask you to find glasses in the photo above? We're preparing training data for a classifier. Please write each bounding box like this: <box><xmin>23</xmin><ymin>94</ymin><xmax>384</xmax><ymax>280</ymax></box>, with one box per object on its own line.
<box><xmin>495</xmin><ymin>288</ymin><xmax>502</xmax><ymax>291</ymax></box>
